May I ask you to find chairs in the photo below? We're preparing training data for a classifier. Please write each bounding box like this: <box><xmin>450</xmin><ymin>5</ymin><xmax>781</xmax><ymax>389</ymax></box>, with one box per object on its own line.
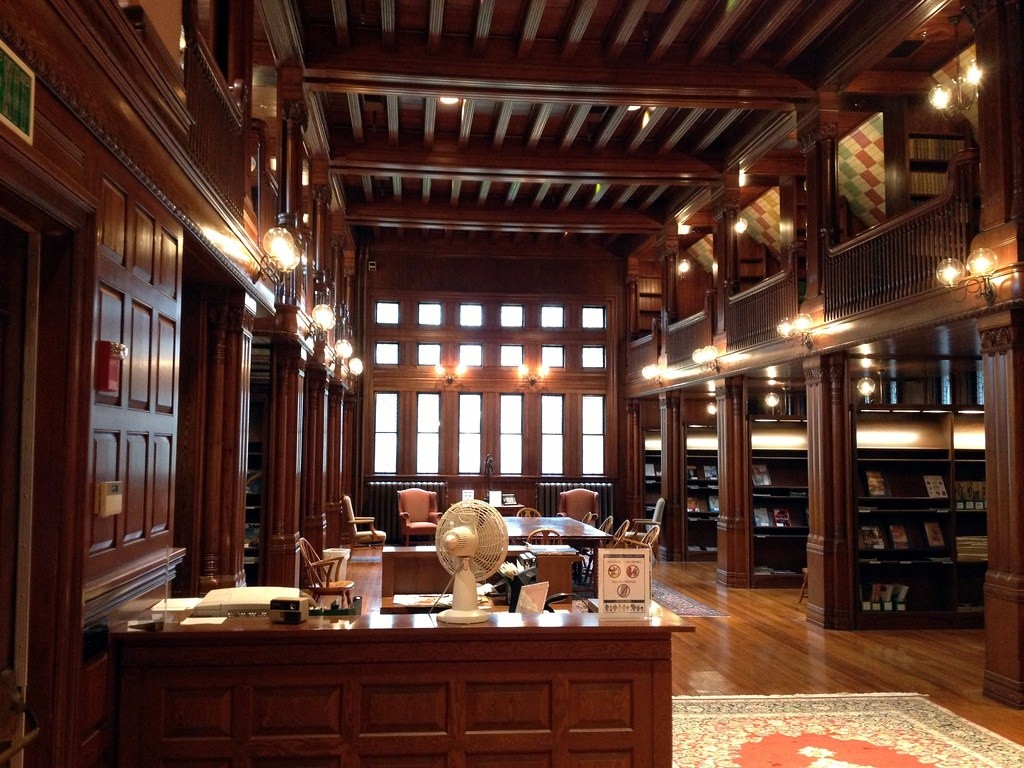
<box><xmin>516</xmin><ymin>508</ymin><xmax>666</xmax><ymax>595</ymax></box>
<box><xmin>299</xmin><ymin>538</ymin><xmax>355</xmax><ymax>607</ymax></box>
<box><xmin>342</xmin><ymin>496</ymin><xmax>386</xmax><ymax>561</ymax></box>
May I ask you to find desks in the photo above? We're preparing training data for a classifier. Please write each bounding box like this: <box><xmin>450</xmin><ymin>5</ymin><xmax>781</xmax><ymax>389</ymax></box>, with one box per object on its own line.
<box><xmin>494</xmin><ymin>503</ymin><xmax>525</xmax><ymax>517</ymax></box>
<box><xmin>109</xmin><ymin>517</ymin><xmax>697</xmax><ymax>768</ymax></box>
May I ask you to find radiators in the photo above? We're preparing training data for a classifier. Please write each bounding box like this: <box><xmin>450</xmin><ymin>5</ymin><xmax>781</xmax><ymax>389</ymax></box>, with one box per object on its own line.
<box><xmin>536</xmin><ymin>482</ymin><xmax>614</xmax><ymax>533</ymax></box>
<box><xmin>369</xmin><ymin>481</ymin><xmax>447</xmax><ymax>543</ymax></box>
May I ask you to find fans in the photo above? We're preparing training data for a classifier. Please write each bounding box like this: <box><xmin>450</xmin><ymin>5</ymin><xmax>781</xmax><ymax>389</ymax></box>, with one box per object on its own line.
<box><xmin>435</xmin><ymin>499</ymin><xmax>509</xmax><ymax>624</ymax></box>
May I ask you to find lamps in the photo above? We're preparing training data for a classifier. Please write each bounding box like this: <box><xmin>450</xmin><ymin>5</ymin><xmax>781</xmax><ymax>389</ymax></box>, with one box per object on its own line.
<box><xmin>692</xmin><ymin>346</ymin><xmax>720</xmax><ymax>372</ymax></box>
<box><xmin>518</xmin><ymin>364</ymin><xmax>550</xmax><ymax>384</ymax></box>
<box><xmin>434</xmin><ymin>364</ymin><xmax>467</xmax><ymax>383</ymax></box>
<box><xmin>928</xmin><ymin>15</ymin><xmax>979</xmax><ymax>120</ymax></box>
<box><xmin>777</xmin><ymin>312</ymin><xmax>815</xmax><ymax>349</ymax></box>
<box><xmin>937</xmin><ymin>247</ymin><xmax>998</xmax><ymax>305</ymax></box>
<box><xmin>707</xmin><ymin>403</ymin><xmax>717</xmax><ymax>414</ymax></box>
<box><xmin>328</xmin><ymin>339</ymin><xmax>363</xmax><ymax>383</ymax></box>
<box><xmin>642</xmin><ymin>365</ymin><xmax>662</xmax><ymax>384</ymax></box>
<box><xmin>304</xmin><ymin>304</ymin><xmax>337</xmax><ymax>341</ymax></box>
<box><xmin>253</xmin><ymin>227</ymin><xmax>301</xmax><ymax>282</ymax></box>
<box><xmin>857</xmin><ymin>376</ymin><xmax>876</xmax><ymax>396</ymax></box>
<box><xmin>764</xmin><ymin>391</ymin><xmax>779</xmax><ymax>407</ymax></box>
<box><xmin>736</xmin><ymin>218</ymin><xmax>748</xmax><ymax>233</ymax></box>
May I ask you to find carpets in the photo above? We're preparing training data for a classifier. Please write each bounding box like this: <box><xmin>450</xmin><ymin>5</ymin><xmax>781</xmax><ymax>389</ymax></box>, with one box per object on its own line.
<box><xmin>651</xmin><ymin>578</ymin><xmax>1024</xmax><ymax>768</ymax></box>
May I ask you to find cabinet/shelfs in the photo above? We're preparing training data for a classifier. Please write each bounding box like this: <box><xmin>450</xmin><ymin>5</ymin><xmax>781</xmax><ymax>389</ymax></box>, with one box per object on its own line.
<box><xmin>645</xmin><ymin>448</ymin><xmax>990</xmax><ymax>630</ymax></box>
<box><xmin>245</xmin><ymin>451</ymin><xmax>263</xmax><ymax>569</ymax></box>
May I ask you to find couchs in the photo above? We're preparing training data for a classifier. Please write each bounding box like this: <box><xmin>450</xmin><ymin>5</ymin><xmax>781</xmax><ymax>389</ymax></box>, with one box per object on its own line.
<box><xmin>558</xmin><ymin>489</ymin><xmax>600</xmax><ymax>528</ymax></box>
<box><xmin>396</xmin><ymin>488</ymin><xmax>443</xmax><ymax>546</ymax></box>
